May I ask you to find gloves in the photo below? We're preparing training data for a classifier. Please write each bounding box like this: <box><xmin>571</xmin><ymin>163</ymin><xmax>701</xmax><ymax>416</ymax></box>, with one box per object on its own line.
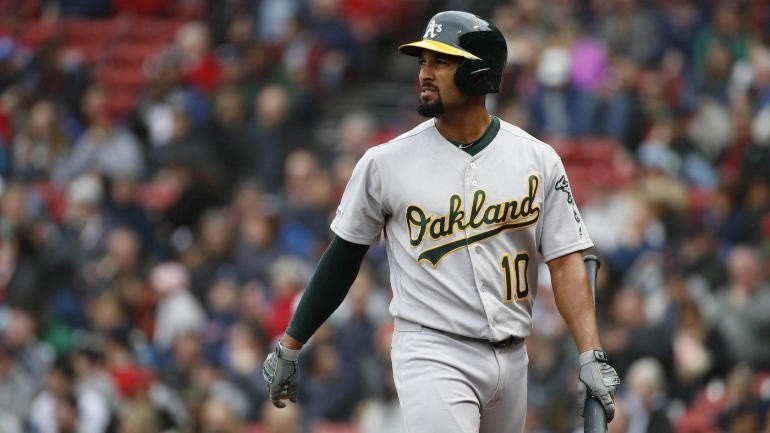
<box><xmin>262</xmin><ymin>342</ymin><xmax>301</xmax><ymax>408</ymax></box>
<box><xmin>575</xmin><ymin>349</ymin><xmax>620</xmax><ymax>424</ymax></box>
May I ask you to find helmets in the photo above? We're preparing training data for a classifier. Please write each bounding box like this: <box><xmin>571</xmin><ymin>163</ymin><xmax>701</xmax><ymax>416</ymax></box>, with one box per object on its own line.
<box><xmin>398</xmin><ymin>10</ymin><xmax>508</xmax><ymax>95</ymax></box>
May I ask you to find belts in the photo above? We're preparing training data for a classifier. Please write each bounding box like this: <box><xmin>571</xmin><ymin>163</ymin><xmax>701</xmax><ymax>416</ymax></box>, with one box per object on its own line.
<box><xmin>421</xmin><ymin>324</ymin><xmax>524</xmax><ymax>349</ymax></box>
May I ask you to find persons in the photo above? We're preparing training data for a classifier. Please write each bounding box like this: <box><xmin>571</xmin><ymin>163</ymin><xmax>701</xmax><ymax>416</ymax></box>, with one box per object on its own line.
<box><xmin>0</xmin><ymin>0</ymin><xmax>770</xmax><ymax>433</ymax></box>
<box><xmin>262</xmin><ymin>10</ymin><xmax>621</xmax><ymax>433</ymax></box>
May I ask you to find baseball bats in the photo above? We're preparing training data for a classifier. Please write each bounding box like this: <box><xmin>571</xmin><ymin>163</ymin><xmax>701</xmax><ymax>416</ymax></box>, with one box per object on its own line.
<box><xmin>582</xmin><ymin>254</ymin><xmax>610</xmax><ymax>433</ymax></box>
